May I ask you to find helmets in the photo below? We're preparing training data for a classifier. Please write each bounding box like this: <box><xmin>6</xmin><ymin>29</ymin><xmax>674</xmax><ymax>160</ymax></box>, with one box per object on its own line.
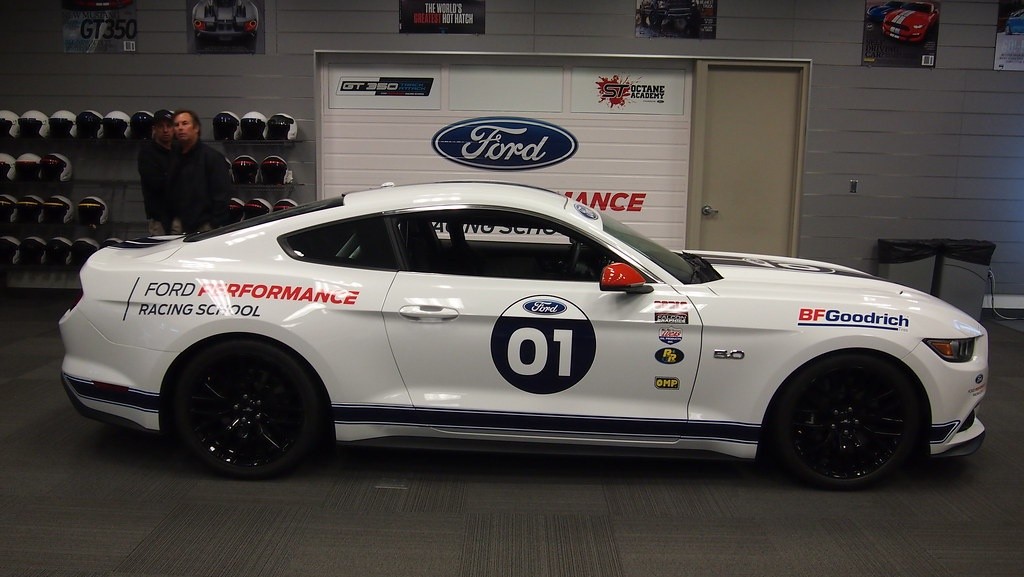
<box><xmin>19</xmin><ymin>235</ymin><xmax>46</xmax><ymax>265</ymax></box>
<box><xmin>231</xmin><ymin>155</ymin><xmax>259</xmax><ymax>185</ymax></box>
<box><xmin>101</xmin><ymin>238</ymin><xmax>123</xmax><ymax>248</ymax></box>
<box><xmin>103</xmin><ymin>111</ymin><xmax>131</xmax><ymax>139</ymax></box>
<box><xmin>0</xmin><ymin>236</ymin><xmax>21</xmax><ymax>265</ymax></box>
<box><xmin>225</xmin><ymin>198</ymin><xmax>245</xmax><ymax>223</ymax></box>
<box><xmin>0</xmin><ymin>152</ymin><xmax>72</xmax><ymax>183</ymax></box>
<box><xmin>78</xmin><ymin>196</ymin><xmax>109</xmax><ymax>225</ymax></box>
<box><xmin>244</xmin><ymin>198</ymin><xmax>273</xmax><ymax>220</ymax></box>
<box><xmin>130</xmin><ymin>111</ymin><xmax>154</xmax><ymax>140</ymax></box>
<box><xmin>72</xmin><ymin>237</ymin><xmax>100</xmax><ymax>266</ymax></box>
<box><xmin>46</xmin><ymin>237</ymin><xmax>72</xmax><ymax>264</ymax></box>
<box><xmin>0</xmin><ymin>193</ymin><xmax>17</xmax><ymax>223</ymax></box>
<box><xmin>40</xmin><ymin>195</ymin><xmax>74</xmax><ymax>225</ymax></box>
<box><xmin>0</xmin><ymin>110</ymin><xmax>20</xmax><ymax>139</ymax></box>
<box><xmin>260</xmin><ymin>155</ymin><xmax>293</xmax><ymax>184</ymax></box>
<box><xmin>212</xmin><ymin>111</ymin><xmax>298</xmax><ymax>140</ymax></box>
<box><xmin>15</xmin><ymin>195</ymin><xmax>44</xmax><ymax>224</ymax></box>
<box><xmin>18</xmin><ymin>110</ymin><xmax>49</xmax><ymax>140</ymax></box>
<box><xmin>76</xmin><ymin>110</ymin><xmax>104</xmax><ymax>139</ymax></box>
<box><xmin>48</xmin><ymin>110</ymin><xmax>77</xmax><ymax>140</ymax></box>
<box><xmin>273</xmin><ymin>199</ymin><xmax>299</xmax><ymax>212</ymax></box>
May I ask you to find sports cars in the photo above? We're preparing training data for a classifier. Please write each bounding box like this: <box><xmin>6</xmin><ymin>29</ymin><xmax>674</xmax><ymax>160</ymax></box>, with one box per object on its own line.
<box><xmin>192</xmin><ymin>0</ymin><xmax>259</xmax><ymax>45</ymax></box>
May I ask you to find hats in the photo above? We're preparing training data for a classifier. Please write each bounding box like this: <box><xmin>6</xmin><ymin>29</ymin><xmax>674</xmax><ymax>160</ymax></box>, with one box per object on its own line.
<box><xmin>153</xmin><ymin>109</ymin><xmax>173</xmax><ymax>121</ymax></box>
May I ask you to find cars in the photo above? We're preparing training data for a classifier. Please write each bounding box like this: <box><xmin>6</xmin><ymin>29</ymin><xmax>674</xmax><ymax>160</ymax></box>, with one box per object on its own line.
<box><xmin>1004</xmin><ymin>8</ymin><xmax>1024</xmax><ymax>35</ymax></box>
<box><xmin>866</xmin><ymin>0</ymin><xmax>939</xmax><ymax>43</ymax></box>
<box><xmin>59</xmin><ymin>173</ymin><xmax>991</xmax><ymax>495</ymax></box>
<box><xmin>639</xmin><ymin>0</ymin><xmax>698</xmax><ymax>28</ymax></box>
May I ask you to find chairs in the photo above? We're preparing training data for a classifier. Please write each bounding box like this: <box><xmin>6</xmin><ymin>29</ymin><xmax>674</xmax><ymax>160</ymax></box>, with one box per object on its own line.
<box><xmin>448</xmin><ymin>223</ymin><xmax>474</xmax><ymax>273</ymax></box>
<box><xmin>421</xmin><ymin>222</ymin><xmax>450</xmax><ymax>271</ymax></box>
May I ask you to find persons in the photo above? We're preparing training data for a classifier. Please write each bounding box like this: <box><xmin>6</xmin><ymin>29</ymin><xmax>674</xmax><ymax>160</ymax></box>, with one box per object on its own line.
<box><xmin>138</xmin><ymin>110</ymin><xmax>183</xmax><ymax>237</ymax></box>
<box><xmin>167</xmin><ymin>110</ymin><xmax>231</xmax><ymax>235</ymax></box>
